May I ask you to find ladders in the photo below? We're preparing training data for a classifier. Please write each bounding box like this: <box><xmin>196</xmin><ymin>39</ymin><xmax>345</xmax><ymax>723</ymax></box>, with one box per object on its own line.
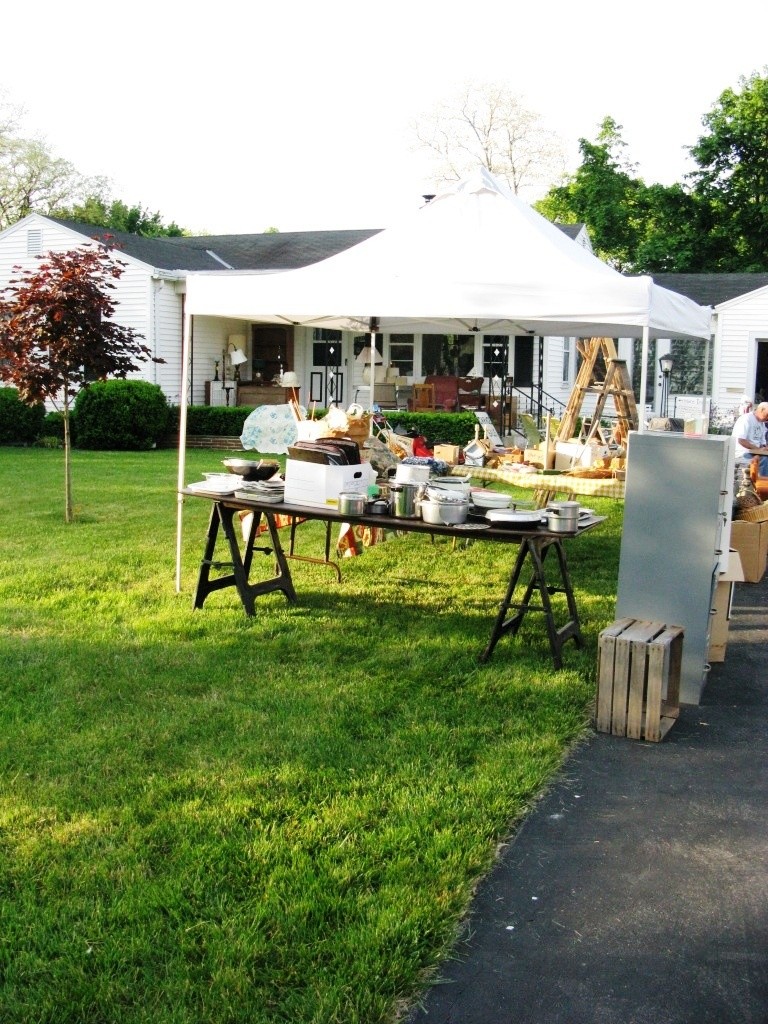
<box><xmin>554</xmin><ymin>337</ymin><xmax>630</xmax><ymax>449</ymax></box>
<box><xmin>586</xmin><ymin>359</ymin><xmax>639</xmax><ymax>443</ymax></box>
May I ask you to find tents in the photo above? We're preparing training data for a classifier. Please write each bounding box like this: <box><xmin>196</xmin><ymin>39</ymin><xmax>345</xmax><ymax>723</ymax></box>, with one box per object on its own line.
<box><xmin>174</xmin><ymin>162</ymin><xmax>711</xmax><ymax>592</ymax></box>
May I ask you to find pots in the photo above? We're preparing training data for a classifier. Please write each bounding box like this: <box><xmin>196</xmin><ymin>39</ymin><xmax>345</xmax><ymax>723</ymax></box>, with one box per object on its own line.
<box><xmin>545</xmin><ymin>500</ymin><xmax>579</xmax><ymax>532</ymax></box>
<box><xmin>222</xmin><ymin>458</ymin><xmax>280</xmax><ymax>481</ymax></box>
<box><xmin>389</xmin><ymin>483</ymin><xmax>422</xmax><ymax>519</ymax></box>
<box><xmin>363</xmin><ymin>493</ymin><xmax>390</xmax><ymax>513</ymax></box>
<box><xmin>484</xmin><ymin>503</ymin><xmax>542</xmax><ymax>530</ymax></box>
<box><xmin>418</xmin><ymin>477</ymin><xmax>471</xmax><ymax>524</ymax></box>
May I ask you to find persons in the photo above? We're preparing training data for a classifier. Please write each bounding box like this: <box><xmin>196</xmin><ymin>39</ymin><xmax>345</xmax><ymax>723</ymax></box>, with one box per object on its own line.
<box><xmin>728</xmin><ymin>402</ymin><xmax>768</xmax><ymax>477</ymax></box>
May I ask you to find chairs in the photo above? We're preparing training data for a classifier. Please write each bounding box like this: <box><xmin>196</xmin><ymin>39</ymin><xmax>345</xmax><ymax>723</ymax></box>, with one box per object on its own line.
<box><xmin>374</xmin><ymin>376</ymin><xmax>486</xmax><ymax>413</ymax></box>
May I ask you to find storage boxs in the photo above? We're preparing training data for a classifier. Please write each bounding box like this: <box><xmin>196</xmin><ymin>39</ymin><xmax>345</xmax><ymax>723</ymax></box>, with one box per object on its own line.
<box><xmin>284</xmin><ymin>458</ymin><xmax>375</xmax><ymax>510</ymax></box>
<box><xmin>595</xmin><ymin>618</ymin><xmax>685</xmax><ymax>742</ymax></box>
<box><xmin>708</xmin><ymin>520</ymin><xmax>768</xmax><ymax>662</ymax></box>
<box><xmin>434</xmin><ymin>419</ymin><xmax>702</xmax><ymax>481</ymax></box>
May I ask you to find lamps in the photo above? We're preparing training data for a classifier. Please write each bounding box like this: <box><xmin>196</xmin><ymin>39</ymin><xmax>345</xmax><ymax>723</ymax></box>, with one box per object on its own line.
<box><xmin>357</xmin><ymin>347</ymin><xmax>384</xmax><ymax>386</ymax></box>
<box><xmin>223</xmin><ymin>343</ymin><xmax>248</xmax><ymax>407</ymax></box>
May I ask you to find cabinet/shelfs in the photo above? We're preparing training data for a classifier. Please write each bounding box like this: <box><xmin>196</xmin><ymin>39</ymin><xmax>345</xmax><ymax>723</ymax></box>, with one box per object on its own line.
<box><xmin>205</xmin><ymin>381</ymin><xmax>237</xmax><ymax>407</ymax></box>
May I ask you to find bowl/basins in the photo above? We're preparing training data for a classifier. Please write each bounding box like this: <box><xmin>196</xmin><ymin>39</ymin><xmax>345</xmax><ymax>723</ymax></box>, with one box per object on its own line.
<box><xmin>201</xmin><ymin>472</ymin><xmax>245</xmax><ymax>488</ymax></box>
<box><xmin>339</xmin><ymin>492</ymin><xmax>364</xmax><ymax>516</ymax></box>
<box><xmin>471</xmin><ymin>492</ymin><xmax>512</xmax><ymax>508</ymax></box>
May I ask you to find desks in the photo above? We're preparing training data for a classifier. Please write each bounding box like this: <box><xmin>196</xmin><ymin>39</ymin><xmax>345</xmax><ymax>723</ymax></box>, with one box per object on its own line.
<box><xmin>176</xmin><ymin>465</ymin><xmax>625</xmax><ymax>672</ymax></box>
